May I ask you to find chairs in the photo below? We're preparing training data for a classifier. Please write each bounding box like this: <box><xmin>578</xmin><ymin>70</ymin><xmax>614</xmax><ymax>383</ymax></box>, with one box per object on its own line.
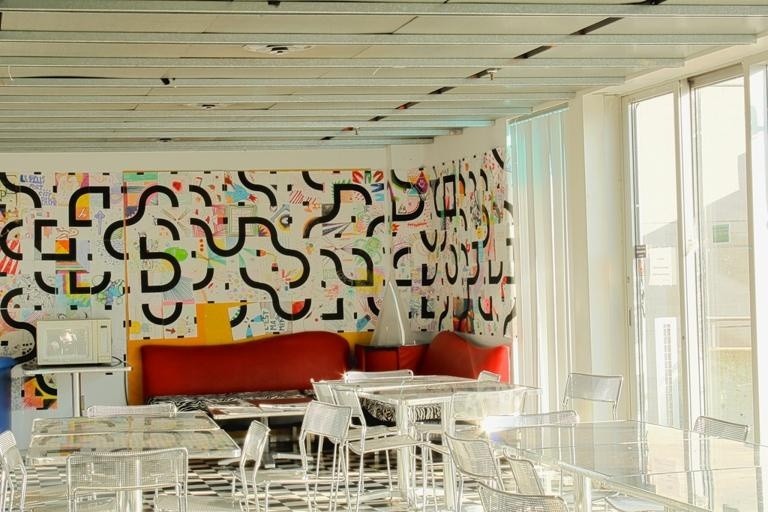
<box><xmin>84</xmin><ymin>402</ymin><xmax>177</xmax><ymax>498</ymax></box>
<box><xmin>422</xmin><ymin>370</ymin><xmax>501</xmax><ymax>486</ymax></box>
<box><xmin>328</xmin><ymin>387</ymin><xmax>431</xmax><ymax>512</ymax></box>
<box><xmin>477</xmin><ymin>481</ymin><xmax>569</xmax><ymax>512</ymax></box>
<box><xmin>66</xmin><ymin>447</ymin><xmax>188</xmax><ymax>512</ymax></box>
<box><xmin>487</xmin><ymin>409</ymin><xmax>580</xmax><ymax>496</ymax></box>
<box><xmin>0</xmin><ymin>430</ymin><xmax>74</xmax><ymax>512</ymax></box>
<box><xmin>562</xmin><ymin>373</ymin><xmax>623</xmax><ymax>421</ymax></box>
<box><xmin>309</xmin><ymin>378</ymin><xmax>394</xmax><ymax>501</ymax></box>
<box><xmin>412</xmin><ymin>387</ymin><xmax>528</xmax><ymax>512</ymax></box>
<box><xmin>604</xmin><ymin>415</ymin><xmax>749</xmax><ymax>511</ymax></box>
<box><xmin>502</xmin><ymin>448</ymin><xmax>545</xmax><ymax>496</ymax></box>
<box><xmin>232</xmin><ymin>400</ymin><xmax>353</xmax><ymax>512</ymax></box>
<box><xmin>444</xmin><ymin>432</ymin><xmax>518</xmax><ymax>512</ymax></box>
<box><xmin>344</xmin><ymin>369</ymin><xmax>413</xmax><ymax>492</ymax></box>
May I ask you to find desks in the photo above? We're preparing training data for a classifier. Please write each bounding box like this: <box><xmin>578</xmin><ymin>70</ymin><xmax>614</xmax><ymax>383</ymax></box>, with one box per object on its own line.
<box><xmin>320</xmin><ymin>375</ymin><xmax>543</xmax><ymax>492</ymax></box>
<box><xmin>22</xmin><ymin>364</ymin><xmax>133</xmax><ymax>417</ymax></box>
<box><xmin>204</xmin><ymin>396</ymin><xmax>318</xmax><ymax>468</ymax></box>
<box><xmin>455</xmin><ymin>419</ymin><xmax>768</xmax><ymax>512</ymax></box>
<box><xmin>25</xmin><ymin>412</ymin><xmax>241</xmax><ymax>512</ymax></box>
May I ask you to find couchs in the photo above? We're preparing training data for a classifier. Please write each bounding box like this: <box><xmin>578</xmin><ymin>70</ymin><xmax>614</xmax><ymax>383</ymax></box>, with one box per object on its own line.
<box><xmin>140</xmin><ymin>331</ymin><xmax>353</xmax><ymax>431</ymax></box>
<box><xmin>356</xmin><ymin>331</ymin><xmax>511</xmax><ymax>422</ymax></box>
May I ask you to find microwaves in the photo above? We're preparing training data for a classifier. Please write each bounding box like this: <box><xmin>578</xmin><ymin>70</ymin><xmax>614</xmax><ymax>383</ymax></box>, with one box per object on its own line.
<box><xmin>36</xmin><ymin>319</ymin><xmax>112</xmax><ymax>366</ymax></box>
